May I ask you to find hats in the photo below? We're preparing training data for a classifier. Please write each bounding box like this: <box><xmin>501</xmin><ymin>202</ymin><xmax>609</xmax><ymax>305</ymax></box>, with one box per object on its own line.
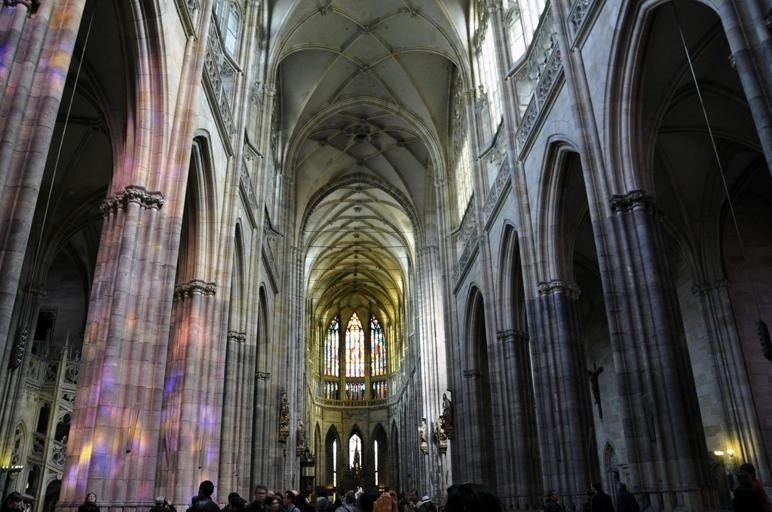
<box><xmin>422</xmin><ymin>495</ymin><xmax>431</xmax><ymax>504</ymax></box>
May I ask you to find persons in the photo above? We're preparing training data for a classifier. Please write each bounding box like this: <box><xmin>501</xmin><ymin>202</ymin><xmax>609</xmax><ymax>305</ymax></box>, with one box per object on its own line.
<box><xmin>438</xmin><ymin>483</ymin><xmax>501</xmax><ymax>511</ymax></box>
<box><xmin>731</xmin><ymin>471</ymin><xmax>762</xmax><ymax>511</ymax></box>
<box><xmin>0</xmin><ymin>492</ymin><xmax>25</xmax><ymax>512</ymax></box>
<box><xmin>78</xmin><ymin>492</ymin><xmax>100</xmax><ymax>512</ymax></box>
<box><xmin>544</xmin><ymin>490</ymin><xmax>562</xmax><ymax>512</ymax></box>
<box><xmin>149</xmin><ymin>496</ymin><xmax>177</xmax><ymax>512</ymax></box>
<box><xmin>221</xmin><ymin>483</ymin><xmax>437</xmax><ymax>511</ymax></box>
<box><xmin>590</xmin><ymin>482</ymin><xmax>615</xmax><ymax>512</ymax></box>
<box><xmin>186</xmin><ymin>481</ymin><xmax>220</xmax><ymax>511</ymax></box>
<box><xmin>740</xmin><ymin>463</ymin><xmax>769</xmax><ymax>511</ymax></box>
<box><xmin>615</xmin><ymin>482</ymin><xmax>640</xmax><ymax>512</ymax></box>
